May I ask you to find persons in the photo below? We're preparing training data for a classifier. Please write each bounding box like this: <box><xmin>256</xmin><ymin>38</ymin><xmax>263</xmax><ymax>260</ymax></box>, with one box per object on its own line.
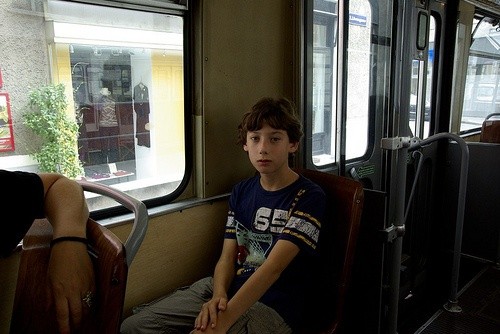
<box><xmin>93</xmin><ymin>87</ymin><xmax>120</xmax><ymax>163</ymax></box>
<box><xmin>0</xmin><ymin>169</ymin><xmax>97</xmax><ymax>334</ymax></box>
<box><xmin>120</xmin><ymin>98</ymin><xmax>336</xmax><ymax>334</ymax></box>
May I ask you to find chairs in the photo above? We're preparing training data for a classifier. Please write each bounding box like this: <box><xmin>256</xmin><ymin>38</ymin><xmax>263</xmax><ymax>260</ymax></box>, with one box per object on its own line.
<box><xmin>292</xmin><ymin>166</ymin><xmax>367</xmax><ymax>334</ymax></box>
<box><xmin>479</xmin><ymin>112</ymin><xmax>500</xmax><ymax>144</ymax></box>
<box><xmin>9</xmin><ymin>180</ymin><xmax>149</xmax><ymax>334</ymax></box>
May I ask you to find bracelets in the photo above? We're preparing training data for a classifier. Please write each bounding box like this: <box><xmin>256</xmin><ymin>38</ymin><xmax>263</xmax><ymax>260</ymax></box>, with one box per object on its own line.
<box><xmin>49</xmin><ymin>236</ymin><xmax>89</xmax><ymax>248</ymax></box>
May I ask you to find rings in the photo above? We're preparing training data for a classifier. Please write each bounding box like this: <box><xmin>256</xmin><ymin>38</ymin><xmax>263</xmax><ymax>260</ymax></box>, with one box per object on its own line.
<box><xmin>80</xmin><ymin>290</ymin><xmax>95</xmax><ymax>308</ymax></box>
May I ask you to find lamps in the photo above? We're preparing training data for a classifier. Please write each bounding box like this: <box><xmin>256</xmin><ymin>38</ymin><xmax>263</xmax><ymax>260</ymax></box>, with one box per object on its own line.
<box><xmin>69</xmin><ymin>44</ymin><xmax>74</xmax><ymax>53</ymax></box>
